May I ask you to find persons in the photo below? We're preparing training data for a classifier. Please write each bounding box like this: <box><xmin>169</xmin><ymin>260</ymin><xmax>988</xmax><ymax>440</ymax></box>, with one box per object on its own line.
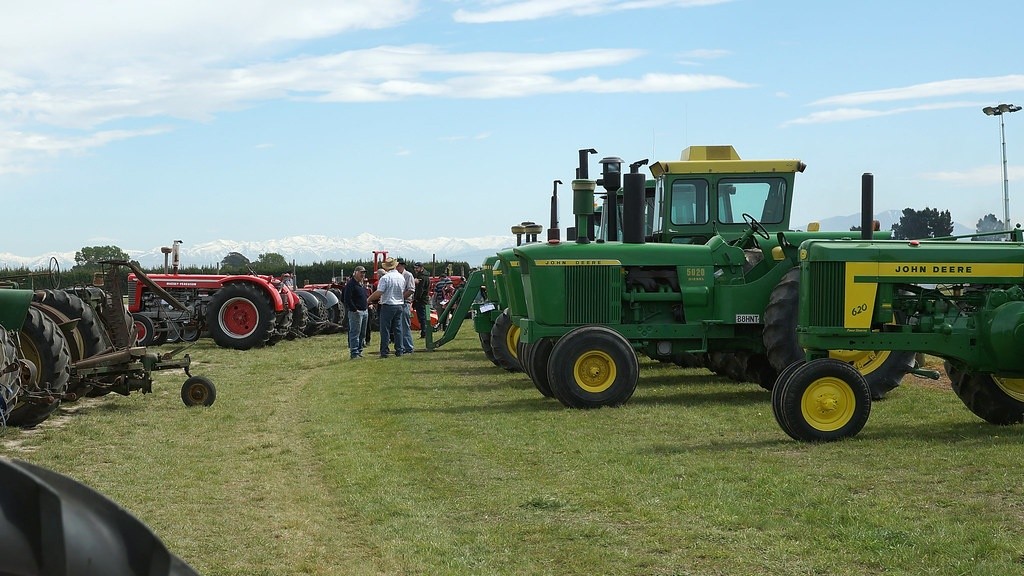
<box><xmin>341</xmin><ymin>257</ymin><xmax>468</xmax><ymax>358</ymax></box>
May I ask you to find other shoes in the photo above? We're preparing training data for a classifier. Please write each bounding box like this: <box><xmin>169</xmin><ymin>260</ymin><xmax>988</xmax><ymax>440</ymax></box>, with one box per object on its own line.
<box><xmin>351</xmin><ymin>354</ymin><xmax>366</xmax><ymax>360</ymax></box>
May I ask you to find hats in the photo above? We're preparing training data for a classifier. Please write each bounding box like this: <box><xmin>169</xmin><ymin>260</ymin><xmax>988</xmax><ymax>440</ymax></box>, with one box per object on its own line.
<box><xmin>446</xmin><ymin>281</ymin><xmax>453</xmax><ymax>284</ymax></box>
<box><xmin>374</xmin><ymin>268</ymin><xmax>385</xmax><ymax>275</ymax></box>
<box><xmin>382</xmin><ymin>257</ymin><xmax>398</xmax><ymax>269</ymax></box>
<box><xmin>398</xmin><ymin>258</ymin><xmax>406</xmax><ymax>265</ymax></box>
<box><xmin>440</xmin><ymin>273</ymin><xmax>446</xmax><ymax>278</ymax></box>
<box><xmin>461</xmin><ymin>277</ymin><xmax>466</xmax><ymax>282</ymax></box>
<box><xmin>355</xmin><ymin>266</ymin><xmax>368</xmax><ymax>272</ymax></box>
<box><xmin>414</xmin><ymin>262</ymin><xmax>423</xmax><ymax>268</ymax></box>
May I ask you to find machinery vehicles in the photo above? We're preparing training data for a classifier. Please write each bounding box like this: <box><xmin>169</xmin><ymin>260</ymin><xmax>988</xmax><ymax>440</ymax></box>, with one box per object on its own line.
<box><xmin>0</xmin><ymin>144</ymin><xmax>942</xmax><ymax>576</ymax></box>
<box><xmin>769</xmin><ymin>171</ymin><xmax>1024</xmax><ymax>444</ymax></box>
